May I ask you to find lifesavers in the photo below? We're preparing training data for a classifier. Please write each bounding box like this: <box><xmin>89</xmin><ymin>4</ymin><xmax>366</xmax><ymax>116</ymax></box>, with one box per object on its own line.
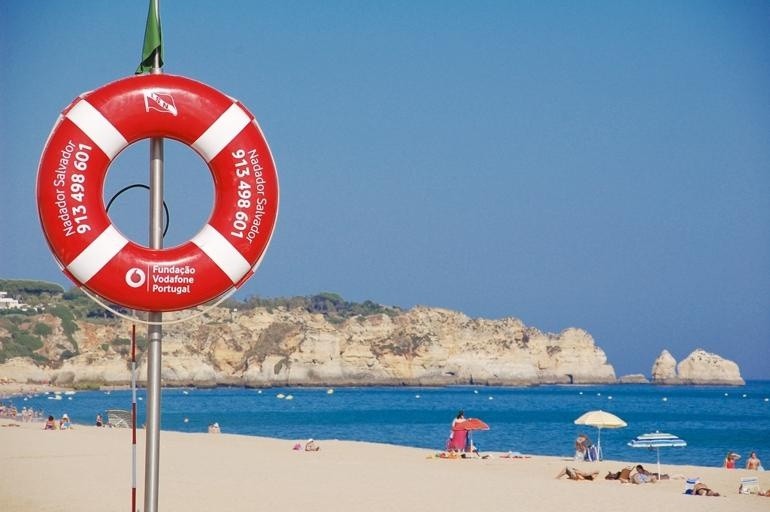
<box><xmin>34</xmin><ymin>76</ymin><xmax>280</xmax><ymax>313</ymax></box>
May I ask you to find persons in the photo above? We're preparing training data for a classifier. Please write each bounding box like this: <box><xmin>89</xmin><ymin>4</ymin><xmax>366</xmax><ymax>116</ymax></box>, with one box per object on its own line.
<box><xmin>0</xmin><ymin>399</ymin><xmax>113</xmax><ymax>431</ymax></box>
<box><xmin>292</xmin><ymin>438</ymin><xmax>320</xmax><ymax>452</ymax></box>
<box><xmin>725</xmin><ymin>451</ymin><xmax>742</xmax><ymax>469</ymax></box>
<box><xmin>689</xmin><ymin>479</ymin><xmax>721</xmax><ymax>496</ymax></box>
<box><xmin>575</xmin><ymin>434</ymin><xmax>597</xmax><ymax>462</ymax></box>
<box><xmin>449</xmin><ymin>410</ymin><xmax>467</xmax><ymax>453</ymax></box>
<box><xmin>746</xmin><ymin>451</ymin><xmax>762</xmax><ymax>470</ymax></box>
<box><xmin>555</xmin><ymin>464</ymin><xmax>600</xmax><ymax>481</ymax></box>
<box><xmin>758</xmin><ymin>489</ymin><xmax>770</xmax><ymax>496</ymax></box>
<box><xmin>208</xmin><ymin>422</ymin><xmax>220</xmax><ymax>433</ymax></box>
<box><xmin>635</xmin><ymin>464</ymin><xmax>670</xmax><ymax>480</ymax></box>
<box><xmin>605</xmin><ymin>470</ymin><xmax>622</xmax><ymax>480</ymax></box>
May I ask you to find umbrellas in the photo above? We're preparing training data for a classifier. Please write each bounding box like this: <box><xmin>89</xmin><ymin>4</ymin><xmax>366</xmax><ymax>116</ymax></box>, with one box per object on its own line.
<box><xmin>573</xmin><ymin>409</ymin><xmax>628</xmax><ymax>461</ymax></box>
<box><xmin>627</xmin><ymin>430</ymin><xmax>688</xmax><ymax>480</ymax></box>
<box><xmin>452</xmin><ymin>417</ymin><xmax>490</xmax><ymax>453</ymax></box>
